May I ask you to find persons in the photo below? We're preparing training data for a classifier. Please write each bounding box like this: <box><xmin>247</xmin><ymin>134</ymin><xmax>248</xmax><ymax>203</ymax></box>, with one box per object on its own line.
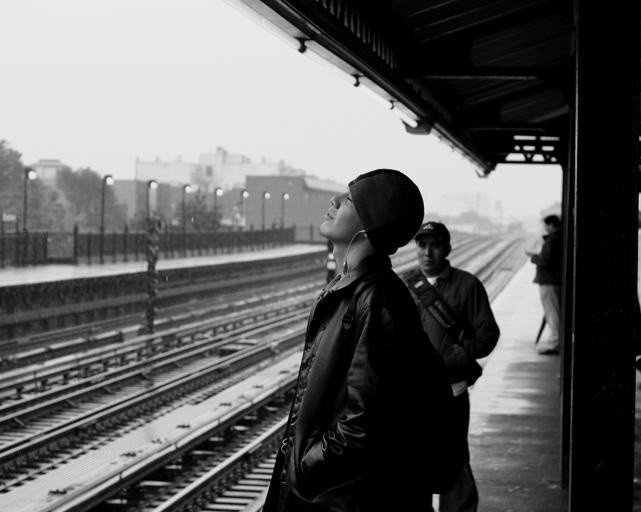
<box><xmin>398</xmin><ymin>221</ymin><xmax>502</xmax><ymax>512</ymax></box>
<box><xmin>266</xmin><ymin>167</ymin><xmax>463</xmax><ymax>512</ymax></box>
<box><xmin>523</xmin><ymin>213</ymin><xmax>563</xmax><ymax>359</ymax></box>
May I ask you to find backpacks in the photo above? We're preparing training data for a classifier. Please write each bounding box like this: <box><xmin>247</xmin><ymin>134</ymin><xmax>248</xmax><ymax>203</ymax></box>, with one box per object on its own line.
<box><xmin>341</xmin><ymin>278</ymin><xmax>470</xmax><ymax>495</ymax></box>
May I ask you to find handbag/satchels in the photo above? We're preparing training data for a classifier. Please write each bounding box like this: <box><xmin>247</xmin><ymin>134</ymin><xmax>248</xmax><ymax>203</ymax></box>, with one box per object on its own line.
<box><xmin>439</xmin><ymin>329</ymin><xmax>482</xmax><ymax>387</ymax></box>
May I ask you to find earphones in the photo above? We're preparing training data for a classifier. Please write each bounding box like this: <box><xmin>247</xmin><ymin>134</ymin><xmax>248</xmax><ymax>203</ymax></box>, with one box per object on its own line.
<box><xmin>362</xmin><ymin>230</ymin><xmax>366</xmax><ymax>233</ymax></box>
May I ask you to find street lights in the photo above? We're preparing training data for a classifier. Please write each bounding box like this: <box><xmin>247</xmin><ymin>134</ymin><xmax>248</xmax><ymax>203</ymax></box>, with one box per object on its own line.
<box><xmin>180</xmin><ymin>183</ymin><xmax>192</xmax><ymax>257</ymax></box>
<box><xmin>98</xmin><ymin>175</ymin><xmax>115</xmax><ymax>262</ymax></box>
<box><xmin>145</xmin><ymin>180</ymin><xmax>159</xmax><ymax>225</ymax></box>
<box><xmin>238</xmin><ymin>189</ymin><xmax>249</xmax><ymax>255</ymax></box>
<box><xmin>21</xmin><ymin>168</ymin><xmax>37</xmax><ymax>265</ymax></box>
<box><xmin>212</xmin><ymin>188</ymin><xmax>224</xmax><ymax>255</ymax></box>
<box><xmin>279</xmin><ymin>193</ymin><xmax>289</xmax><ymax>247</ymax></box>
<box><xmin>260</xmin><ymin>191</ymin><xmax>271</xmax><ymax>249</ymax></box>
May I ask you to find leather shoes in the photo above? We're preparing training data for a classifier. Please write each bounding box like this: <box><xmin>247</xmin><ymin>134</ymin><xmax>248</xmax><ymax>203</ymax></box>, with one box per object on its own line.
<box><xmin>540</xmin><ymin>349</ymin><xmax>559</xmax><ymax>355</ymax></box>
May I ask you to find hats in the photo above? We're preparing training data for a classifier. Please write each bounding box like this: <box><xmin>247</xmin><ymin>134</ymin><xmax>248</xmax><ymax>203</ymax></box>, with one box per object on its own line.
<box><xmin>348</xmin><ymin>169</ymin><xmax>424</xmax><ymax>259</ymax></box>
<box><xmin>414</xmin><ymin>222</ymin><xmax>450</xmax><ymax>245</ymax></box>
